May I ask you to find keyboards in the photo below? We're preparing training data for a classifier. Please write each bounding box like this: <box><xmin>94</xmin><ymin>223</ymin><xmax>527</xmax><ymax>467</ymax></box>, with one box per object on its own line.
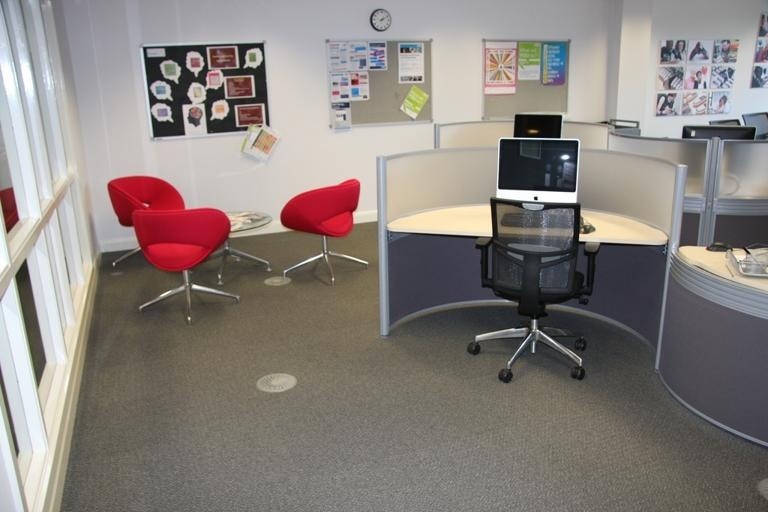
<box><xmin>501</xmin><ymin>212</ymin><xmax>583</xmax><ymax>229</ymax></box>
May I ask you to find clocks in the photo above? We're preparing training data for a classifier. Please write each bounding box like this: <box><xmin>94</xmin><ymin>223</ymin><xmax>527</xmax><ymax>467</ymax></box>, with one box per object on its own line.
<box><xmin>370</xmin><ymin>7</ymin><xmax>392</xmax><ymax>31</ymax></box>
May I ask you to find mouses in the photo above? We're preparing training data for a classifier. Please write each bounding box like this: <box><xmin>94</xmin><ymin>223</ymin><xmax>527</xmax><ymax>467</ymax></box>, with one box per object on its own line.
<box><xmin>707</xmin><ymin>242</ymin><xmax>732</xmax><ymax>252</ymax></box>
<box><xmin>580</xmin><ymin>224</ymin><xmax>595</xmax><ymax>234</ymax></box>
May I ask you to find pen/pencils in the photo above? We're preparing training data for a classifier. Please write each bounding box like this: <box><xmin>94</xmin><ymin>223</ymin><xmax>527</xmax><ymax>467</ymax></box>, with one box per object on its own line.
<box><xmin>742</xmin><ymin>245</ymin><xmax>767</xmax><ymax>274</ymax></box>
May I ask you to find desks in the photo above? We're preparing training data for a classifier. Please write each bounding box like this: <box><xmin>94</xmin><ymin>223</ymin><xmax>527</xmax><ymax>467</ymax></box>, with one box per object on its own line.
<box><xmin>200</xmin><ymin>209</ymin><xmax>272</xmax><ymax>287</ymax></box>
<box><xmin>659</xmin><ymin>244</ymin><xmax>767</xmax><ymax>448</ymax></box>
<box><xmin>376</xmin><ymin>200</ymin><xmax>670</xmax><ymax>372</ymax></box>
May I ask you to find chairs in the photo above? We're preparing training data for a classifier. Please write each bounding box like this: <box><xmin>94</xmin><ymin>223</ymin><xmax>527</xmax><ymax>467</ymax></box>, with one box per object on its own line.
<box><xmin>466</xmin><ymin>199</ymin><xmax>600</xmax><ymax>384</ymax></box>
<box><xmin>707</xmin><ymin>111</ymin><xmax>768</xmax><ymax>142</ymax></box>
<box><xmin>280</xmin><ymin>178</ymin><xmax>371</xmax><ymax>284</ymax></box>
<box><xmin>105</xmin><ymin>176</ymin><xmax>239</xmax><ymax>325</ymax></box>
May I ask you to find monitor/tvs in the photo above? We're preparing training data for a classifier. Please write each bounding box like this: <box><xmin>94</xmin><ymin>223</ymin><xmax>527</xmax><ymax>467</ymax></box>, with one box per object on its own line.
<box><xmin>710</xmin><ymin>119</ymin><xmax>740</xmax><ymax>126</ymax></box>
<box><xmin>742</xmin><ymin>112</ymin><xmax>768</xmax><ymax>139</ymax></box>
<box><xmin>681</xmin><ymin>124</ymin><xmax>756</xmax><ymax>140</ymax></box>
<box><xmin>513</xmin><ymin>112</ymin><xmax>564</xmax><ymax>138</ymax></box>
<box><xmin>496</xmin><ymin>137</ymin><xmax>580</xmax><ymax>204</ymax></box>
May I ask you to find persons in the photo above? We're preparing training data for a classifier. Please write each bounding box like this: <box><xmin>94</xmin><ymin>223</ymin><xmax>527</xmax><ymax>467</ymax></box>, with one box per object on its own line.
<box><xmin>656</xmin><ymin>14</ymin><xmax>768</xmax><ymax>116</ymax></box>
